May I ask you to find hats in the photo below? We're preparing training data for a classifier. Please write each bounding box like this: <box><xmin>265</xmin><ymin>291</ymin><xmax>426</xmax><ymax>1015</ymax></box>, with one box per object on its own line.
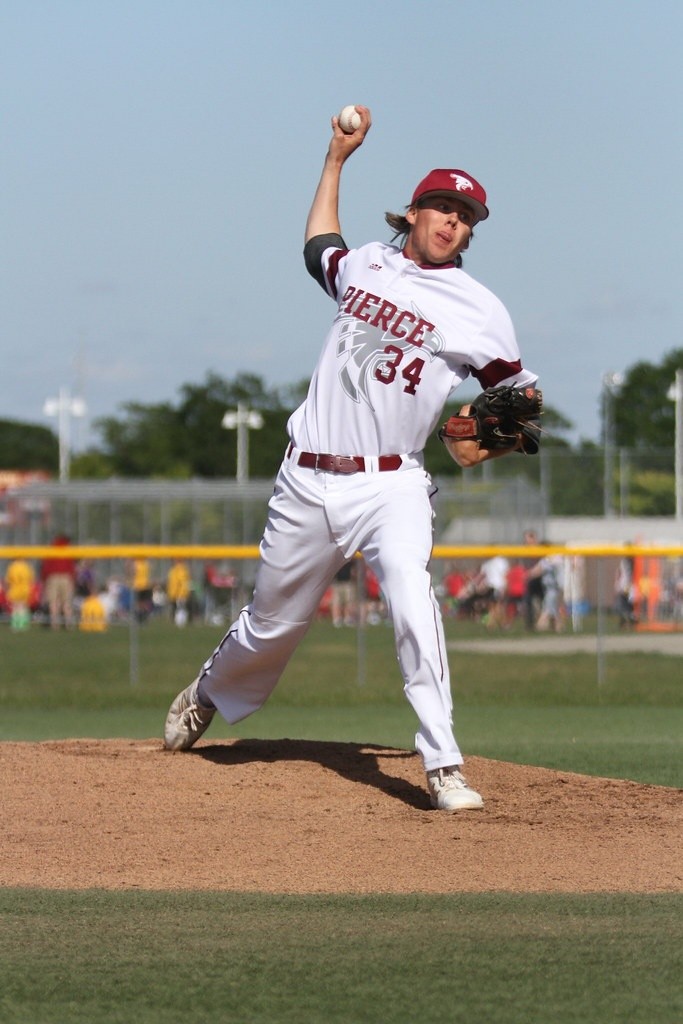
<box><xmin>408</xmin><ymin>169</ymin><xmax>490</xmax><ymax>220</ymax></box>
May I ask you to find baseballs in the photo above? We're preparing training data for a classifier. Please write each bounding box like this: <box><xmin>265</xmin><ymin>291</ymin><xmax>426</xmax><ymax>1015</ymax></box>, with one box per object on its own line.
<box><xmin>338</xmin><ymin>105</ymin><xmax>362</xmax><ymax>132</ymax></box>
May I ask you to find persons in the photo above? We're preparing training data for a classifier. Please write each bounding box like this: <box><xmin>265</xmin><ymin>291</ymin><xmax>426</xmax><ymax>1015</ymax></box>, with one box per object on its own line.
<box><xmin>5</xmin><ymin>526</ymin><xmax>681</xmax><ymax>641</ymax></box>
<box><xmin>160</xmin><ymin>102</ymin><xmax>542</xmax><ymax>811</ymax></box>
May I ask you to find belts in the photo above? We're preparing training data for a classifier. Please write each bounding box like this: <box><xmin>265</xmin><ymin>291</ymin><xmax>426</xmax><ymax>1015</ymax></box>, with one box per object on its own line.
<box><xmin>287</xmin><ymin>442</ymin><xmax>426</xmax><ymax>472</ymax></box>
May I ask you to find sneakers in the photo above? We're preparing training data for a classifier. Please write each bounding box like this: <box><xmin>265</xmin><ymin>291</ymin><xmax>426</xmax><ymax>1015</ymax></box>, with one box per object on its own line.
<box><xmin>165</xmin><ymin>669</ymin><xmax>216</xmax><ymax>750</ymax></box>
<box><xmin>426</xmin><ymin>768</ymin><xmax>483</xmax><ymax>810</ymax></box>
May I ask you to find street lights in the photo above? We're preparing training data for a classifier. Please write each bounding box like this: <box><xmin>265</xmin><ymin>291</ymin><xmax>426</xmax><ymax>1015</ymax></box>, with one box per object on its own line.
<box><xmin>44</xmin><ymin>387</ymin><xmax>87</xmax><ymax>487</ymax></box>
<box><xmin>601</xmin><ymin>373</ymin><xmax>625</xmax><ymax>519</ymax></box>
<box><xmin>666</xmin><ymin>369</ymin><xmax>683</xmax><ymax>521</ymax></box>
<box><xmin>221</xmin><ymin>403</ymin><xmax>264</xmax><ymax>482</ymax></box>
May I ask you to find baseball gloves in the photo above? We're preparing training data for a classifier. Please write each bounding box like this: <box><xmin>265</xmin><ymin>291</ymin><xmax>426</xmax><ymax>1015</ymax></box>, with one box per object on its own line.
<box><xmin>438</xmin><ymin>381</ymin><xmax>542</xmax><ymax>468</ymax></box>
